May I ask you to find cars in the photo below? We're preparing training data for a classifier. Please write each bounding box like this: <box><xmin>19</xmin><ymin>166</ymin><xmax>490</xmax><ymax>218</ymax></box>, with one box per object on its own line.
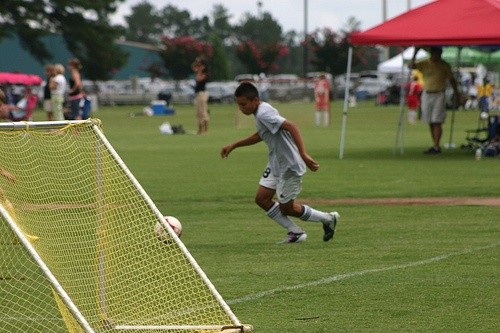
<box><xmin>82</xmin><ymin>70</ymin><xmax>395</xmax><ymax>105</ymax></box>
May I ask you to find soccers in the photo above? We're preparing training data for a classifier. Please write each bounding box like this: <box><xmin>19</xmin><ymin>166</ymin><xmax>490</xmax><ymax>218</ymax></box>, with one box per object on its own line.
<box><xmin>153</xmin><ymin>214</ymin><xmax>183</xmax><ymax>244</ymax></box>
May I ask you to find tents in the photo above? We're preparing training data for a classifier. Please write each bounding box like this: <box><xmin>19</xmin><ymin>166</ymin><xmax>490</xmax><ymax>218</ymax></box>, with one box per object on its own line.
<box><xmin>377</xmin><ymin>48</ymin><xmax>429</xmax><ymax>108</ymax></box>
<box><xmin>339</xmin><ymin>0</ymin><xmax>500</xmax><ymax>163</ymax></box>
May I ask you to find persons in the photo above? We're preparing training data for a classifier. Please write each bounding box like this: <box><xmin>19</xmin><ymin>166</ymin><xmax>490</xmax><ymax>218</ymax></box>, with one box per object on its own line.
<box><xmin>219</xmin><ymin>82</ymin><xmax>341</xmax><ymax>244</ymax></box>
<box><xmin>0</xmin><ymin>46</ymin><xmax>500</xmax><ymax>241</ymax></box>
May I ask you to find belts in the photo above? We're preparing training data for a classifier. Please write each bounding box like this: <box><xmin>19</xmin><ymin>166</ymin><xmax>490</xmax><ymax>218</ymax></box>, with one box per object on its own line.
<box><xmin>426</xmin><ymin>89</ymin><xmax>445</xmax><ymax>94</ymax></box>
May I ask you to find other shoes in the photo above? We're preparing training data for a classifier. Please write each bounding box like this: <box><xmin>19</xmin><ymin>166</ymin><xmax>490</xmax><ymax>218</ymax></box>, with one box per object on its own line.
<box><xmin>424</xmin><ymin>147</ymin><xmax>441</xmax><ymax>155</ymax></box>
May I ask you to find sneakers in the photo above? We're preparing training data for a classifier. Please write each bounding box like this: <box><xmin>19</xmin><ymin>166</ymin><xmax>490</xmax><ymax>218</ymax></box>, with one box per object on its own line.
<box><xmin>323</xmin><ymin>211</ymin><xmax>340</xmax><ymax>241</ymax></box>
<box><xmin>278</xmin><ymin>231</ymin><xmax>309</xmax><ymax>244</ymax></box>
